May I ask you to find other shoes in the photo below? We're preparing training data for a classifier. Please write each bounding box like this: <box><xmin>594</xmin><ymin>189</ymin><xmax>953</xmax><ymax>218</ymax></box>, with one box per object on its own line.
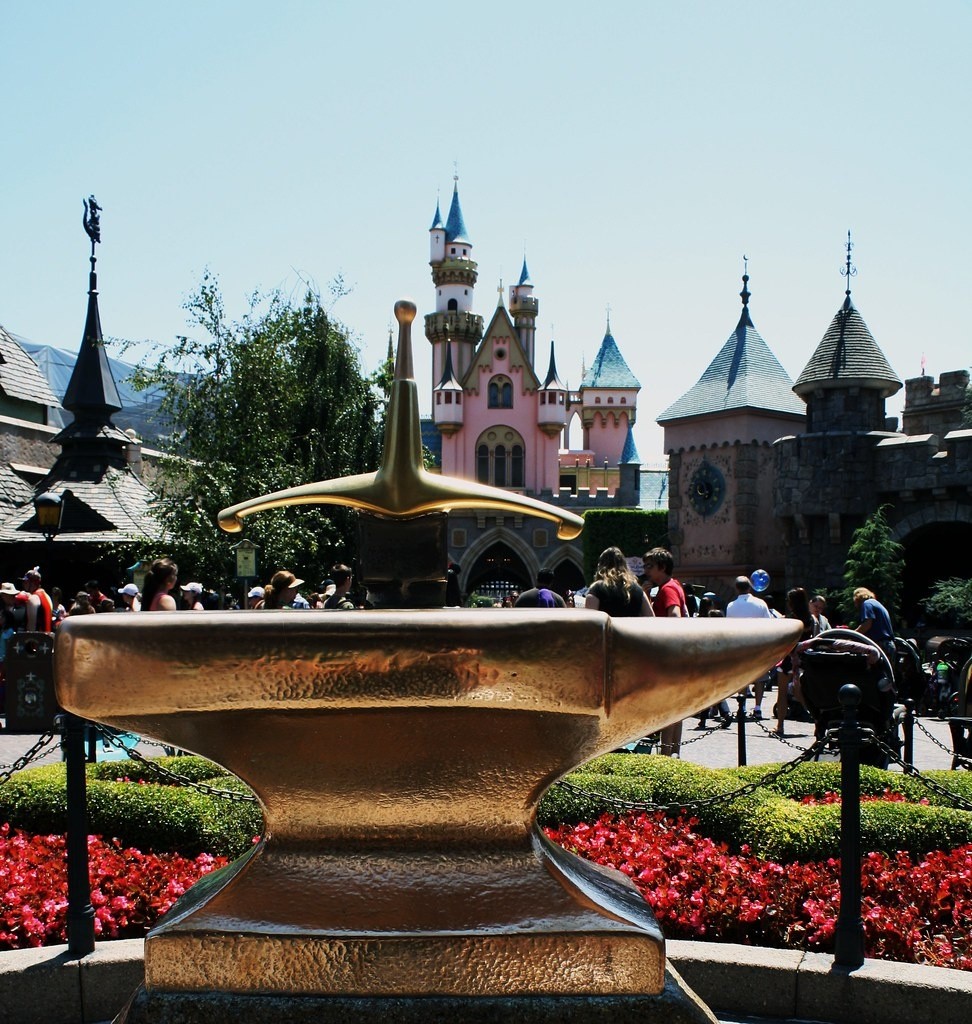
<box><xmin>753</xmin><ymin>710</ymin><xmax>762</xmax><ymax>720</ymax></box>
<box><xmin>720</xmin><ymin>713</ymin><xmax>732</xmax><ymax>728</ymax></box>
<box><xmin>737</xmin><ymin>710</ymin><xmax>747</xmax><ymax>716</ymax></box>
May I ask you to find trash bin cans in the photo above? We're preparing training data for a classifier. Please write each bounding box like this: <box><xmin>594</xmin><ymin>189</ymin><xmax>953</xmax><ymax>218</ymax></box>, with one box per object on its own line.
<box><xmin>925</xmin><ymin>635</ymin><xmax>970</xmax><ymax>716</ymax></box>
<box><xmin>3</xmin><ymin>630</ymin><xmax>60</xmax><ymax>734</ymax></box>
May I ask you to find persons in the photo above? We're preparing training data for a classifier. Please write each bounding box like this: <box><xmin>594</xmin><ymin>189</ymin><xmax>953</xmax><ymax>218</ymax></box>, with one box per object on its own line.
<box><xmin>322</xmin><ymin>564</ymin><xmax>354</xmax><ymax>610</ymax></box>
<box><xmin>726</xmin><ymin>575</ymin><xmax>772</xmax><ymax>618</ymax></box>
<box><xmin>642</xmin><ymin>546</ymin><xmax>690</xmax><ymax>757</ymax></box>
<box><xmin>585</xmin><ymin>546</ymin><xmax>657</xmax><ymax>617</ymax></box>
<box><xmin>853</xmin><ymin>587</ymin><xmax>896</xmax><ymax>666</ymax></box>
<box><xmin>17</xmin><ymin>565</ymin><xmax>53</xmax><ymax>632</ymax></box>
<box><xmin>514</xmin><ymin>567</ymin><xmax>567</xmax><ymax>608</ymax></box>
<box><xmin>0</xmin><ymin>546</ymin><xmax>896</xmax><ymax>738</ymax></box>
<box><xmin>263</xmin><ymin>571</ymin><xmax>305</xmax><ymax>610</ymax></box>
<box><xmin>140</xmin><ymin>557</ymin><xmax>178</xmax><ymax>611</ymax></box>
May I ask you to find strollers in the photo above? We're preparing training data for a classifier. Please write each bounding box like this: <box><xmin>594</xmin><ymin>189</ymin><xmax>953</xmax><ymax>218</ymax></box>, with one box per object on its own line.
<box><xmin>800</xmin><ymin>626</ymin><xmax>901</xmax><ymax>775</ymax></box>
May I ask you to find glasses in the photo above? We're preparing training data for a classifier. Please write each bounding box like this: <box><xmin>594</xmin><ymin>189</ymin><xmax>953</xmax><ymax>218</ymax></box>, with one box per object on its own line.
<box><xmin>510</xmin><ymin>596</ymin><xmax>517</xmax><ymax>599</ymax></box>
<box><xmin>51</xmin><ymin>592</ymin><xmax>58</xmax><ymax>597</ymax></box>
<box><xmin>643</xmin><ymin>563</ymin><xmax>654</xmax><ymax>570</ymax></box>
<box><xmin>785</xmin><ymin>598</ymin><xmax>790</xmax><ymax>602</ymax></box>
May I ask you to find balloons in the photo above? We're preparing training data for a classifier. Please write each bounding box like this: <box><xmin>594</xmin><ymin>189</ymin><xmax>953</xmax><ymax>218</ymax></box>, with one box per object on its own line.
<box><xmin>749</xmin><ymin>569</ymin><xmax>770</xmax><ymax>592</ymax></box>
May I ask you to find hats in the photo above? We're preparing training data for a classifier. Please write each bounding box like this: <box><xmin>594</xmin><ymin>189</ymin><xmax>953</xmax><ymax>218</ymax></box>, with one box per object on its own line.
<box><xmin>248</xmin><ymin>587</ymin><xmax>265</xmax><ymax>598</ymax></box>
<box><xmin>118</xmin><ymin>584</ymin><xmax>139</xmax><ymax>596</ymax></box>
<box><xmin>325</xmin><ymin>585</ymin><xmax>336</xmax><ymax>595</ymax></box>
<box><xmin>180</xmin><ymin>582</ymin><xmax>202</xmax><ymax>593</ymax></box>
<box><xmin>18</xmin><ymin>570</ymin><xmax>42</xmax><ymax>581</ymax></box>
<box><xmin>0</xmin><ymin>583</ymin><xmax>20</xmax><ymax>595</ymax></box>
<box><xmin>77</xmin><ymin>591</ymin><xmax>90</xmax><ymax>597</ymax></box>
<box><xmin>318</xmin><ymin>578</ymin><xmax>334</xmax><ymax>589</ymax></box>
<box><xmin>272</xmin><ymin>571</ymin><xmax>305</xmax><ymax>588</ymax></box>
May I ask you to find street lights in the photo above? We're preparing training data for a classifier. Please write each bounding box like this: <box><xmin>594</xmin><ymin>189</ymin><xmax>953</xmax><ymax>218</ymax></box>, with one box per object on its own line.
<box><xmin>32</xmin><ymin>491</ymin><xmax>63</xmax><ymax>634</ymax></box>
